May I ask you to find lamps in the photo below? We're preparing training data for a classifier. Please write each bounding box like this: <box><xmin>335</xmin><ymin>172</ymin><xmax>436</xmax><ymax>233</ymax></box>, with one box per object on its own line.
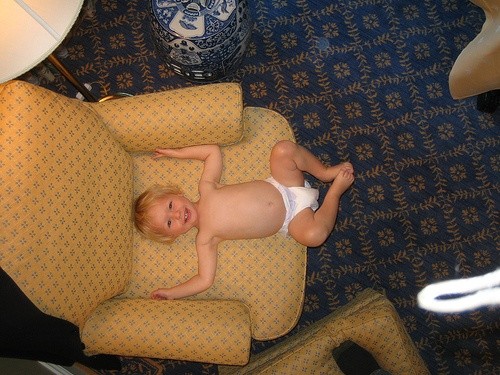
<box><xmin>1</xmin><ymin>0</ymin><xmax>134</xmax><ymax>104</ymax></box>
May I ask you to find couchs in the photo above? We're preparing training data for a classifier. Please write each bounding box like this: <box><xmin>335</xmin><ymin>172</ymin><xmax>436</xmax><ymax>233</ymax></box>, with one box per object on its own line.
<box><xmin>1</xmin><ymin>78</ymin><xmax>306</xmax><ymax>365</ymax></box>
<box><xmin>235</xmin><ymin>287</ymin><xmax>434</xmax><ymax>375</ymax></box>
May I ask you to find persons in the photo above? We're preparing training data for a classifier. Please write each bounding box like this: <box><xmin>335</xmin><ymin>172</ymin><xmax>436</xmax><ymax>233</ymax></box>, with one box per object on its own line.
<box><xmin>133</xmin><ymin>141</ymin><xmax>355</xmax><ymax>301</ymax></box>
